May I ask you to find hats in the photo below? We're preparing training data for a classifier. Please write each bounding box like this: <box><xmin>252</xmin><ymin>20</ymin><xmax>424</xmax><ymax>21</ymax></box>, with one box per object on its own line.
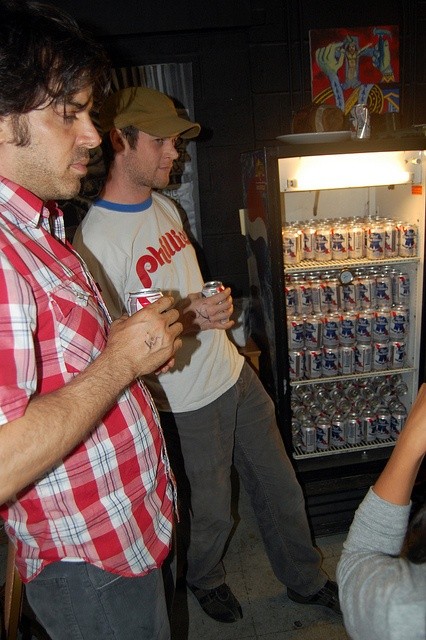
<box><xmin>99</xmin><ymin>85</ymin><xmax>201</xmax><ymax>140</ymax></box>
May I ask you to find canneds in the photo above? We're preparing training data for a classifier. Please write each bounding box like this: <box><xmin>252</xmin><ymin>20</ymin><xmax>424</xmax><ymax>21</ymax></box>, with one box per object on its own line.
<box><xmin>373</xmin><ymin>344</ymin><xmax>393</xmax><ymax>371</ymax></box>
<box><xmin>289</xmin><ymin>350</ymin><xmax>305</xmax><ymax>383</ymax></box>
<box><xmin>292</xmin><ymin>375</ymin><xmax>408</xmax><ymax>419</ymax></box>
<box><xmin>306</xmin><ymin>352</ymin><xmax>322</xmax><ymax>380</ymax></box>
<box><xmin>339</xmin><ymin>344</ymin><xmax>356</xmax><ymax>375</ymax></box>
<box><xmin>317</xmin><ymin>415</ymin><xmax>333</xmax><ymax>447</ymax></box>
<box><xmin>292</xmin><ymin>422</ymin><xmax>301</xmax><ymax>447</ymax></box>
<box><xmin>321</xmin><ymin>349</ymin><xmax>341</xmax><ymax>377</ymax></box>
<box><xmin>376</xmin><ymin>409</ymin><xmax>392</xmax><ymax>441</ymax></box>
<box><xmin>345</xmin><ymin>415</ymin><xmax>363</xmax><ymax>445</ymax></box>
<box><xmin>282</xmin><ymin>214</ymin><xmax>418</xmax><ymax>266</ymax></box>
<box><xmin>391</xmin><ymin>339</ymin><xmax>407</xmax><ymax>369</ymax></box>
<box><xmin>353</xmin><ymin>345</ymin><xmax>374</xmax><ymax>373</ymax></box>
<box><xmin>363</xmin><ymin>413</ymin><xmax>378</xmax><ymax>442</ymax></box>
<box><xmin>330</xmin><ymin>416</ymin><xmax>347</xmax><ymax>447</ymax></box>
<box><xmin>285</xmin><ymin>267</ymin><xmax>411</xmax><ymax>316</ymax></box>
<box><xmin>128</xmin><ymin>286</ymin><xmax>166</xmax><ymax>318</ymax></box>
<box><xmin>289</xmin><ymin>306</ymin><xmax>410</xmax><ymax>350</ymax></box>
<box><xmin>302</xmin><ymin>421</ymin><xmax>318</xmax><ymax>453</ymax></box>
<box><xmin>202</xmin><ymin>281</ymin><xmax>230</xmax><ymax>325</ymax></box>
<box><xmin>391</xmin><ymin>408</ymin><xmax>409</xmax><ymax>439</ymax></box>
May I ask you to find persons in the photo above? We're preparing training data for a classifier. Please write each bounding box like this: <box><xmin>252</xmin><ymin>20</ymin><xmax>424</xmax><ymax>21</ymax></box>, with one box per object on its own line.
<box><xmin>0</xmin><ymin>0</ymin><xmax>183</xmax><ymax>639</ymax></box>
<box><xmin>72</xmin><ymin>86</ymin><xmax>337</xmax><ymax>623</ymax></box>
<box><xmin>337</xmin><ymin>381</ymin><xmax>426</xmax><ymax>638</ymax></box>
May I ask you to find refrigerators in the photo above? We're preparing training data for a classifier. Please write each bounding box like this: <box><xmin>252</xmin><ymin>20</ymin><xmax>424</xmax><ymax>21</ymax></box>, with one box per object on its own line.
<box><xmin>242</xmin><ymin>134</ymin><xmax>425</xmax><ymax>539</ymax></box>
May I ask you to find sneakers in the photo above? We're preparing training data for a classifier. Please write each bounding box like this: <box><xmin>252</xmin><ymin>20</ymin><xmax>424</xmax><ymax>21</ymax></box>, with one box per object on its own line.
<box><xmin>190</xmin><ymin>582</ymin><xmax>243</xmax><ymax>624</ymax></box>
<box><xmin>286</xmin><ymin>581</ymin><xmax>343</xmax><ymax>614</ymax></box>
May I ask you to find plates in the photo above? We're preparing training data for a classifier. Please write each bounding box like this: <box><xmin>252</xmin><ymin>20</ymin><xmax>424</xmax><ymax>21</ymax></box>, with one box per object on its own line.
<box><xmin>279</xmin><ymin>131</ymin><xmax>351</xmax><ymax>143</ymax></box>
